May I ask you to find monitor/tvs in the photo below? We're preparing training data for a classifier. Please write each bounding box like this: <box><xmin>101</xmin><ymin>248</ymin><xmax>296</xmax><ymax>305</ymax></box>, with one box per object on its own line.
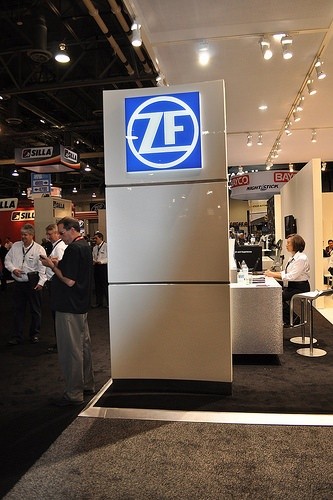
<box><xmin>285</xmin><ymin>215</ymin><xmax>297</xmax><ymax>239</ymax></box>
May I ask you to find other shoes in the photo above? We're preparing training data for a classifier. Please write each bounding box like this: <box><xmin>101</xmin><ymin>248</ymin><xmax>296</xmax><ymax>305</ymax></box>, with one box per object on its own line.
<box><xmin>83</xmin><ymin>390</ymin><xmax>95</xmax><ymax>396</ymax></box>
<box><xmin>48</xmin><ymin>392</ymin><xmax>85</xmax><ymax>407</ymax></box>
<box><xmin>46</xmin><ymin>343</ymin><xmax>58</xmax><ymax>354</ymax></box>
<box><xmin>91</xmin><ymin>304</ymin><xmax>103</xmax><ymax>308</ymax></box>
<box><xmin>282</xmin><ymin>316</ymin><xmax>300</xmax><ymax>328</ymax></box>
<box><xmin>8</xmin><ymin>334</ymin><xmax>25</xmax><ymax>345</ymax></box>
<box><xmin>31</xmin><ymin>336</ymin><xmax>40</xmax><ymax>344</ymax></box>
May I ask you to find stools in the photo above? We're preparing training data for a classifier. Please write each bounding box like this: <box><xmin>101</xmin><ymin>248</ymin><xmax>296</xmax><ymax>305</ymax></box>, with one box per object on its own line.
<box><xmin>290</xmin><ymin>289</ymin><xmax>333</xmax><ymax>358</ymax></box>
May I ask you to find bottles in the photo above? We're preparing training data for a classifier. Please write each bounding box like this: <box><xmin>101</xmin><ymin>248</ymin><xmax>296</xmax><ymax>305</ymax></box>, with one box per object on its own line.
<box><xmin>237</xmin><ymin>260</ymin><xmax>248</xmax><ymax>284</ymax></box>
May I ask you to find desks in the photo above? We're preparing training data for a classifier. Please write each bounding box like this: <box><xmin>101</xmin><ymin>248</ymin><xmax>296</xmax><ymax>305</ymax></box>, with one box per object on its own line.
<box><xmin>262</xmin><ymin>256</ymin><xmax>275</xmax><ymax>271</ymax></box>
<box><xmin>228</xmin><ymin>271</ymin><xmax>284</xmax><ymax>366</ymax></box>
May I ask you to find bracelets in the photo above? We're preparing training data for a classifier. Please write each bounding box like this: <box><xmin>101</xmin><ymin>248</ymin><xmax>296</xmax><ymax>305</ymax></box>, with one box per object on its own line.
<box><xmin>52</xmin><ymin>265</ymin><xmax>56</xmax><ymax>271</ymax></box>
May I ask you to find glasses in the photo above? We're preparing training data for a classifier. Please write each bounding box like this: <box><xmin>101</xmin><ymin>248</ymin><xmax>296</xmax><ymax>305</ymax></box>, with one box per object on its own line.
<box><xmin>57</xmin><ymin>230</ymin><xmax>66</xmax><ymax>237</ymax></box>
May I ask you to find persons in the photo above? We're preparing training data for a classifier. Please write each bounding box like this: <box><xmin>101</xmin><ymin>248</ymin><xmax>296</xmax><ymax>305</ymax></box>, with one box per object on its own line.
<box><xmin>40</xmin><ymin>216</ymin><xmax>95</xmax><ymax>407</ymax></box>
<box><xmin>229</xmin><ymin>227</ymin><xmax>333</xmax><ymax>285</ymax></box>
<box><xmin>0</xmin><ymin>223</ymin><xmax>108</xmax><ymax>352</ymax></box>
<box><xmin>264</xmin><ymin>234</ymin><xmax>310</xmax><ymax>328</ymax></box>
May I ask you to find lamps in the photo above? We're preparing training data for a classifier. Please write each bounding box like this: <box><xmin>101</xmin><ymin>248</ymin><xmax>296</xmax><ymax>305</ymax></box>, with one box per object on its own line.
<box><xmin>237</xmin><ymin>77</ymin><xmax>326</xmax><ymax>175</ymax></box>
<box><xmin>129</xmin><ymin>22</ymin><xmax>142</xmax><ymax>48</ymax></box>
<box><xmin>313</xmin><ymin>59</ymin><xmax>326</xmax><ymax>81</ymax></box>
<box><xmin>22</xmin><ymin>191</ymin><xmax>27</xmax><ymax>195</ymax></box>
<box><xmin>84</xmin><ymin>162</ymin><xmax>92</xmax><ymax>172</ymax></box>
<box><xmin>53</xmin><ymin>44</ymin><xmax>71</xmax><ymax>63</ymax></box>
<box><xmin>156</xmin><ymin>76</ymin><xmax>166</xmax><ymax>87</ymax></box>
<box><xmin>258</xmin><ymin>35</ymin><xmax>272</xmax><ymax>61</ymax></box>
<box><xmin>73</xmin><ymin>187</ymin><xmax>77</xmax><ymax>192</ymax></box>
<box><xmin>91</xmin><ymin>192</ymin><xmax>96</xmax><ymax>197</ymax></box>
<box><xmin>12</xmin><ymin>168</ymin><xmax>20</xmax><ymax>177</ymax></box>
<box><xmin>279</xmin><ymin>35</ymin><xmax>293</xmax><ymax>60</ymax></box>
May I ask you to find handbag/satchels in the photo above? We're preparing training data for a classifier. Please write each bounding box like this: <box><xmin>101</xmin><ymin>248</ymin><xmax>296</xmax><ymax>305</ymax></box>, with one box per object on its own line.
<box><xmin>27</xmin><ymin>271</ymin><xmax>40</xmax><ymax>289</ymax></box>
